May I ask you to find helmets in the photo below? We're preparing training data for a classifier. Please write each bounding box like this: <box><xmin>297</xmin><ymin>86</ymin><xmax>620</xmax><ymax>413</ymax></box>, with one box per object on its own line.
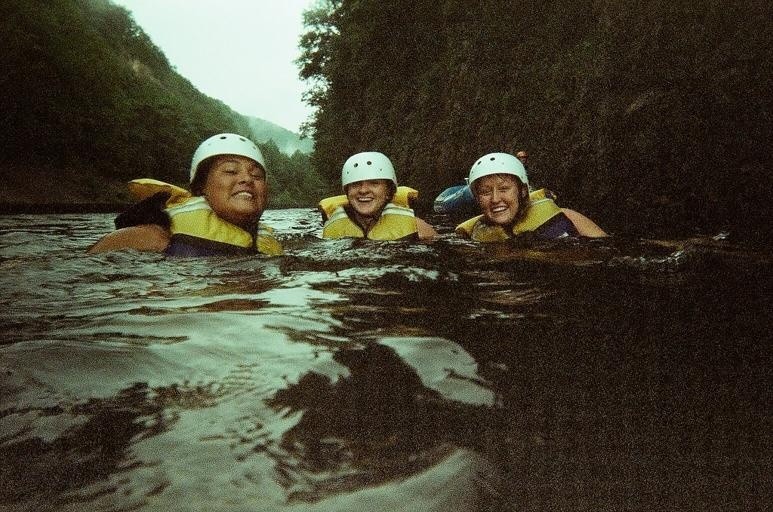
<box><xmin>468</xmin><ymin>152</ymin><xmax>530</xmax><ymax>200</ymax></box>
<box><xmin>342</xmin><ymin>151</ymin><xmax>398</xmax><ymax>193</ymax></box>
<box><xmin>189</xmin><ymin>132</ymin><xmax>268</xmax><ymax>185</ymax></box>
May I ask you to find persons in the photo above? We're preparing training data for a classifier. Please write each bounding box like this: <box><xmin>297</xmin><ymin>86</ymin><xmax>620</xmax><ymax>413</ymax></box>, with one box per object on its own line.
<box><xmin>318</xmin><ymin>152</ymin><xmax>439</xmax><ymax>240</ymax></box>
<box><xmin>455</xmin><ymin>151</ymin><xmax>609</xmax><ymax>243</ymax></box>
<box><xmin>84</xmin><ymin>132</ymin><xmax>286</xmax><ymax>257</ymax></box>
<box><xmin>515</xmin><ymin>150</ymin><xmax>535</xmax><ymax>186</ymax></box>
